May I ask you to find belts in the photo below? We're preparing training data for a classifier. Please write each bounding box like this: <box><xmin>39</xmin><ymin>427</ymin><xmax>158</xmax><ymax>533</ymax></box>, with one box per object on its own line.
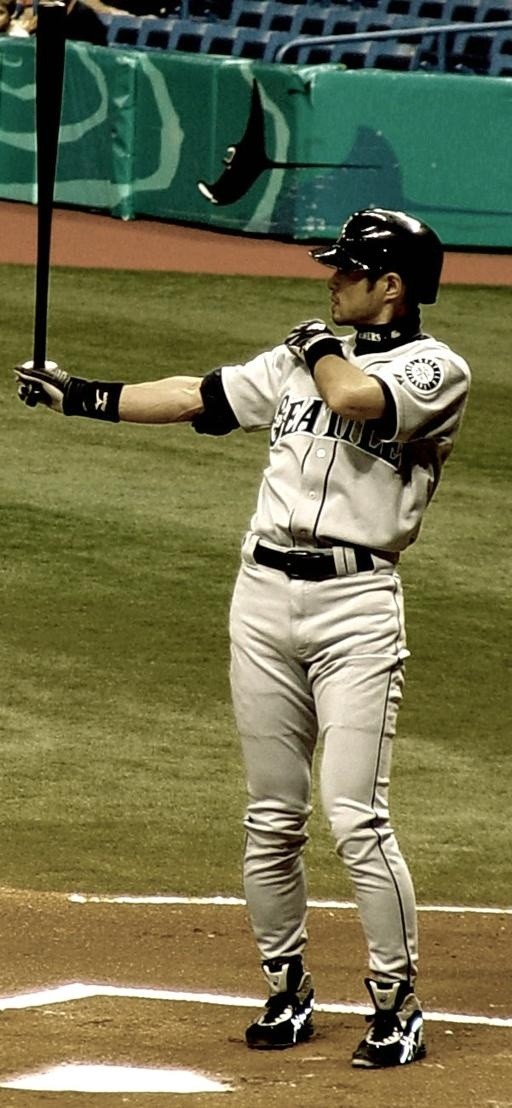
<box><xmin>252</xmin><ymin>544</ymin><xmax>376</xmax><ymax>581</ymax></box>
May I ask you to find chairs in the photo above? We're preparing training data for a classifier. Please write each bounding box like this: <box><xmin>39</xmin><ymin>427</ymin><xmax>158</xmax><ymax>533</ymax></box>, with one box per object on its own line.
<box><xmin>108</xmin><ymin>0</ymin><xmax>512</xmax><ymax>79</ymax></box>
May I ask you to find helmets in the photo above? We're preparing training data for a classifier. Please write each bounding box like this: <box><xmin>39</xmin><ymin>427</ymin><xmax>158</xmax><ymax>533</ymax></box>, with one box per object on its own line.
<box><xmin>305</xmin><ymin>205</ymin><xmax>447</xmax><ymax>305</ymax></box>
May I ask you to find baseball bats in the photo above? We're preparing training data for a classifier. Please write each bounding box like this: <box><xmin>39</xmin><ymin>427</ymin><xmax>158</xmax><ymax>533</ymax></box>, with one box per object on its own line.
<box><xmin>27</xmin><ymin>4</ymin><xmax>69</xmax><ymax>403</ymax></box>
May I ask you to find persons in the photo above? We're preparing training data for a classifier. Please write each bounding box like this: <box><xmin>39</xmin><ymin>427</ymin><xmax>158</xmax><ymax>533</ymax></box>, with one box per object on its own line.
<box><xmin>15</xmin><ymin>203</ymin><xmax>473</xmax><ymax>1070</ymax></box>
<box><xmin>0</xmin><ymin>0</ymin><xmax>234</xmax><ymax>48</ymax></box>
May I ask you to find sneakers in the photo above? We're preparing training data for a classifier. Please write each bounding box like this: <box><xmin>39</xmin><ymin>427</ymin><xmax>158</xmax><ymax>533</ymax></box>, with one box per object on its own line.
<box><xmin>350</xmin><ymin>978</ymin><xmax>426</xmax><ymax>1071</ymax></box>
<box><xmin>245</xmin><ymin>955</ymin><xmax>316</xmax><ymax>1051</ymax></box>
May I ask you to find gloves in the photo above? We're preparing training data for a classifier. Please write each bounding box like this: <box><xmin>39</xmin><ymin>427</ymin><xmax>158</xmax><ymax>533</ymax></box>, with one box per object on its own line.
<box><xmin>13</xmin><ymin>356</ymin><xmax>123</xmax><ymax>425</ymax></box>
<box><xmin>284</xmin><ymin>316</ymin><xmax>347</xmax><ymax>378</ymax></box>
